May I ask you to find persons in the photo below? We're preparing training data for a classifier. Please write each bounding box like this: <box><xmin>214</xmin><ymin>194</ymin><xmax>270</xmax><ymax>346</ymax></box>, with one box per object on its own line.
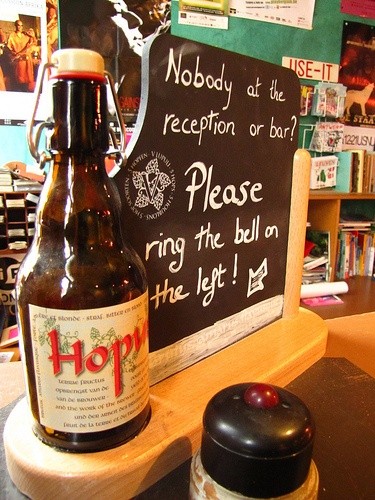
<box><xmin>7</xmin><ymin>16</ymin><xmax>40</xmax><ymax>92</ymax></box>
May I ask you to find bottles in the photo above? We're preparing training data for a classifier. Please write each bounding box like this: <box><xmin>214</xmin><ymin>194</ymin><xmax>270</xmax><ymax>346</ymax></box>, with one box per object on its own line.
<box><xmin>13</xmin><ymin>47</ymin><xmax>152</xmax><ymax>455</ymax></box>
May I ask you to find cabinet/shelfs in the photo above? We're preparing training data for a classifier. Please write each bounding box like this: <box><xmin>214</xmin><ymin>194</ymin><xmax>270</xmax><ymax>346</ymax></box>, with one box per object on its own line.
<box><xmin>0</xmin><ymin>190</ymin><xmax>42</xmax><ymax>254</ymax></box>
<box><xmin>301</xmin><ymin>187</ymin><xmax>375</xmax><ymax>282</ymax></box>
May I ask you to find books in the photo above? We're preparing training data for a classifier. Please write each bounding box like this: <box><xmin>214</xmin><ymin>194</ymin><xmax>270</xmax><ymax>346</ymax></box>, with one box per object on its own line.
<box><xmin>299</xmin><ymin>75</ymin><xmax>375</xmax><ymax>307</ymax></box>
<box><xmin>0</xmin><ymin>192</ymin><xmax>42</xmax><ymax>255</ymax></box>
<box><xmin>0</xmin><ymin>167</ymin><xmax>44</xmax><ymax>193</ymax></box>
